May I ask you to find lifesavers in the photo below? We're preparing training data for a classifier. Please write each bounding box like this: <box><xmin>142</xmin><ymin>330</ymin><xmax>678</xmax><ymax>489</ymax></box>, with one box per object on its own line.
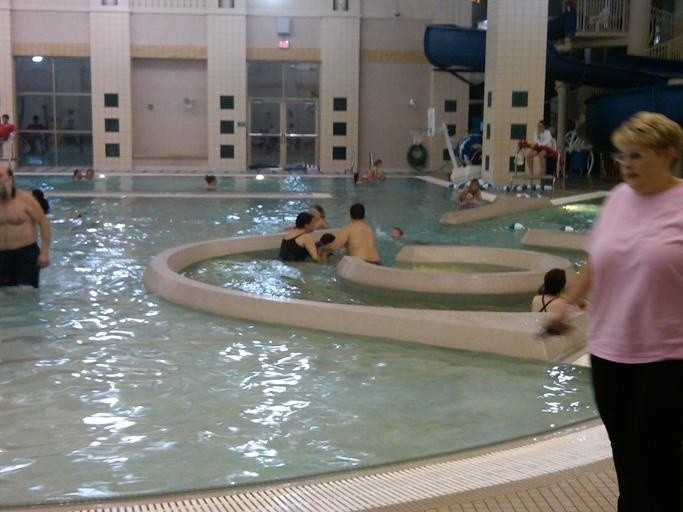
<box><xmin>459</xmin><ymin>135</ymin><xmax>482</xmax><ymax>166</ymax></box>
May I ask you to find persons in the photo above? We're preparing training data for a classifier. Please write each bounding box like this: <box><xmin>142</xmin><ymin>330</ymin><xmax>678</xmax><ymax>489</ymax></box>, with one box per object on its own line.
<box><xmin>63</xmin><ymin>108</ymin><xmax>81</xmax><ymax>133</ymax></box>
<box><xmin>30</xmin><ymin>187</ymin><xmax>49</xmax><ymax>216</ymax></box>
<box><xmin>309</xmin><ymin>205</ymin><xmax>331</xmax><ymax>229</ymax></box>
<box><xmin>304</xmin><ymin>233</ymin><xmax>335</xmax><ymax>260</ymax></box>
<box><xmin>564</xmin><ymin>109</ymin><xmax>682</xmax><ymax>511</ymax></box>
<box><xmin>453</xmin><ymin>178</ymin><xmax>481</xmax><ymax>208</ymax></box>
<box><xmin>353</xmin><ymin>171</ymin><xmax>363</xmax><ymax>184</ymax></box>
<box><xmin>526</xmin><ymin>119</ymin><xmax>552</xmax><ymax>176</ymax></box>
<box><xmin>23</xmin><ymin>115</ymin><xmax>46</xmax><ymax>155</ymax></box>
<box><xmin>0</xmin><ymin>113</ymin><xmax>16</xmax><ymax>158</ymax></box>
<box><xmin>71</xmin><ymin>168</ymin><xmax>80</xmax><ymax>183</ymax></box>
<box><xmin>390</xmin><ymin>226</ymin><xmax>403</xmax><ymax>239</ymax></box>
<box><xmin>251</xmin><ymin>107</ymin><xmax>309</xmax><ymax>160</ymax></box>
<box><xmin>367</xmin><ymin>158</ymin><xmax>385</xmax><ymax>185</ymax></box>
<box><xmin>317</xmin><ymin>202</ymin><xmax>384</xmax><ymax>265</ymax></box>
<box><xmin>587</xmin><ymin>2</ymin><xmax>612</xmax><ymax>30</ymax></box>
<box><xmin>203</xmin><ymin>174</ymin><xmax>217</xmax><ymax>193</ymax></box>
<box><xmin>78</xmin><ymin>168</ymin><xmax>95</xmax><ymax>183</ymax></box>
<box><xmin>278</xmin><ymin>212</ymin><xmax>321</xmax><ymax>264</ymax></box>
<box><xmin>0</xmin><ymin>160</ymin><xmax>51</xmax><ymax>290</ymax></box>
<box><xmin>530</xmin><ymin>266</ymin><xmax>594</xmax><ymax>338</ymax></box>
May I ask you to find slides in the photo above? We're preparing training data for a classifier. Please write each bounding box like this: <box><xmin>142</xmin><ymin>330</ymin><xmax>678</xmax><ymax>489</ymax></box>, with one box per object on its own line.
<box><xmin>423</xmin><ymin>10</ymin><xmax>683</xmax><ymax>157</ymax></box>
